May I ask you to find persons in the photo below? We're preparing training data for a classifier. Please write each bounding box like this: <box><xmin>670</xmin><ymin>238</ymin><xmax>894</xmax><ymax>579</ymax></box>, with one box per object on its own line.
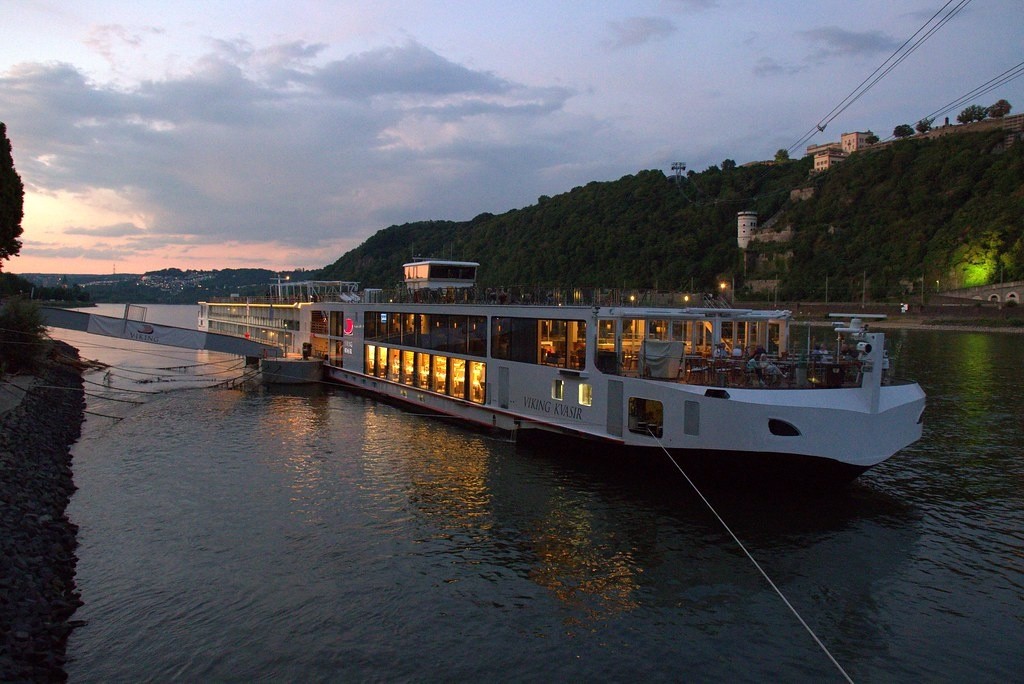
<box><xmin>714</xmin><ymin>340</ymin><xmax>860</xmax><ymax>387</ymax></box>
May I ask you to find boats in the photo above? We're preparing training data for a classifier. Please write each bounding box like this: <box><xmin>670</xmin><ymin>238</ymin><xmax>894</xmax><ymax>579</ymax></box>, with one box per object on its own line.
<box><xmin>198</xmin><ymin>260</ymin><xmax>927</xmax><ymax>485</ymax></box>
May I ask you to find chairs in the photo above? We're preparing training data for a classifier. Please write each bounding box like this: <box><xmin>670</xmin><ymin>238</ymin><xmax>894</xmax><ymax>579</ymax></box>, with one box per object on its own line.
<box><xmin>541</xmin><ymin>339</ymin><xmax>863</xmax><ymax>390</ymax></box>
